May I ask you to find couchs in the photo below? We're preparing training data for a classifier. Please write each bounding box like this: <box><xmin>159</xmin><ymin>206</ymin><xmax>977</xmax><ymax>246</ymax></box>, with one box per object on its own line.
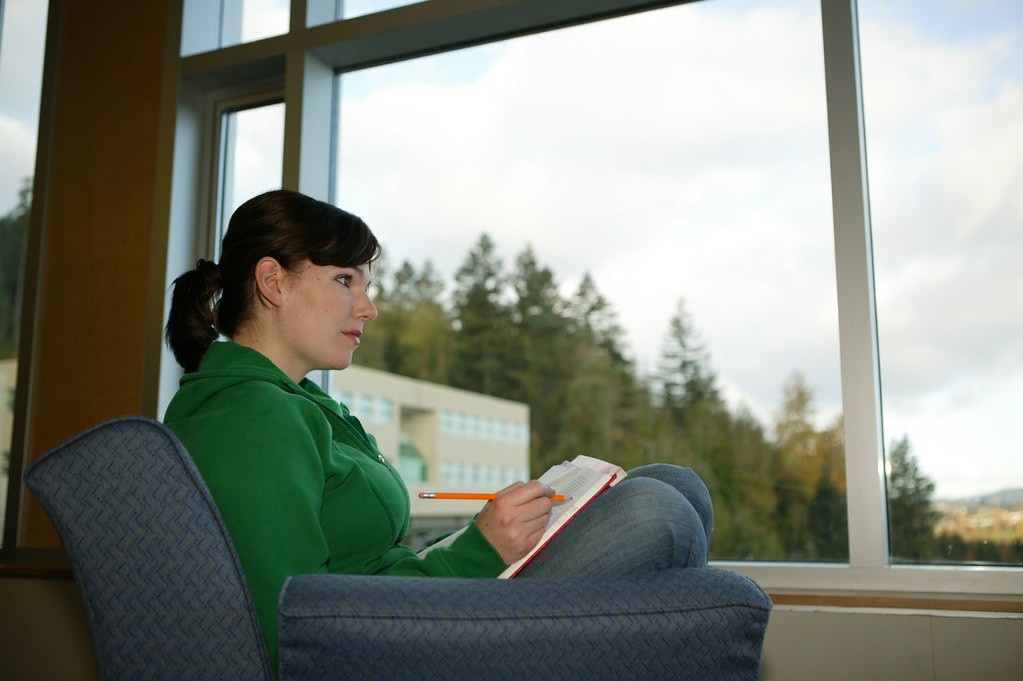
<box><xmin>23</xmin><ymin>417</ymin><xmax>773</xmax><ymax>681</ymax></box>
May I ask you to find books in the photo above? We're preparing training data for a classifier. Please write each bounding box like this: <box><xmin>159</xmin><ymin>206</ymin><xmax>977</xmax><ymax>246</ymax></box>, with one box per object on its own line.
<box><xmin>418</xmin><ymin>455</ymin><xmax>629</xmax><ymax>580</ymax></box>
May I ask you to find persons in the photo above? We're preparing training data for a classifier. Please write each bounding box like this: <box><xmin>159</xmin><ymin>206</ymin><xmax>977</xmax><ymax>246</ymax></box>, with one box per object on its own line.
<box><xmin>163</xmin><ymin>189</ymin><xmax>715</xmax><ymax>681</ymax></box>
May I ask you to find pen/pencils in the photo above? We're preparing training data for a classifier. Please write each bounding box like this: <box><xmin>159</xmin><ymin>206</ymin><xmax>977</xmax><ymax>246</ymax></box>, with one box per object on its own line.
<box><xmin>418</xmin><ymin>490</ymin><xmax>574</xmax><ymax>505</ymax></box>
